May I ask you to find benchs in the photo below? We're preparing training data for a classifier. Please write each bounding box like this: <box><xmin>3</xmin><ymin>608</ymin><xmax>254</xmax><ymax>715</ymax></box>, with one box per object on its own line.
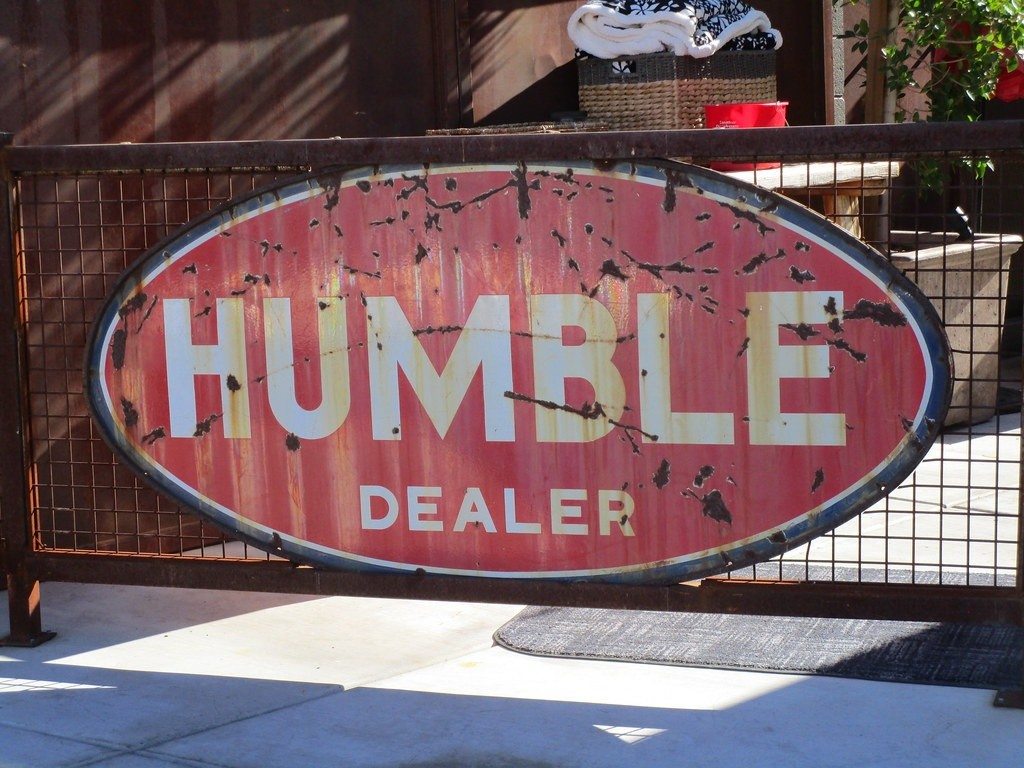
<box><xmin>721</xmin><ymin>161</ymin><xmax>899</xmax><ymax>242</ymax></box>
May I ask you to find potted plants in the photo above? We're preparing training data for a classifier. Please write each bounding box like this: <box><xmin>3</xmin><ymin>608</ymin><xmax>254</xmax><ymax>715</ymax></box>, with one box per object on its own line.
<box><xmin>832</xmin><ymin>0</ymin><xmax>1024</xmax><ymax>430</ymax></box>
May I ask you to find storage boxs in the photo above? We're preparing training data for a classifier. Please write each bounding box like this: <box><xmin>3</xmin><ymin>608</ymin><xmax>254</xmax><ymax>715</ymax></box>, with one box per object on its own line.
<box><xmin>576</xmin><ymin>48</ymin><xmax>778</xmax><ymax>169</ymax></box>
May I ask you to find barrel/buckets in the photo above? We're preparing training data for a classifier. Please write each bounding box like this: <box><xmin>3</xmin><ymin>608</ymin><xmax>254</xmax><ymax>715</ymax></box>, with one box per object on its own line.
<box><xmin>702</xmin><ymin>101</ymin><xmax>791</xmax><ymax>171</ymax></box>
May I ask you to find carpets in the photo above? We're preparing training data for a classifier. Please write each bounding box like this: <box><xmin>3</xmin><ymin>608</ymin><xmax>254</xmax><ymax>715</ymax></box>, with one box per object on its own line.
<box><xmin>492</xmin><ymin>563</ymin><xmax>1024</xmax><ymax>690</ymax></box>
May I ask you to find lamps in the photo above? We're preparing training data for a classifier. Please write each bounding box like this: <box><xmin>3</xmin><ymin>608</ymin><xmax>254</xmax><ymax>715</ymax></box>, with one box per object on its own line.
<box><xmin>945</xmin><ymin>204</ymin><xmax>973</xmax><ymax>242</ymax></box>
